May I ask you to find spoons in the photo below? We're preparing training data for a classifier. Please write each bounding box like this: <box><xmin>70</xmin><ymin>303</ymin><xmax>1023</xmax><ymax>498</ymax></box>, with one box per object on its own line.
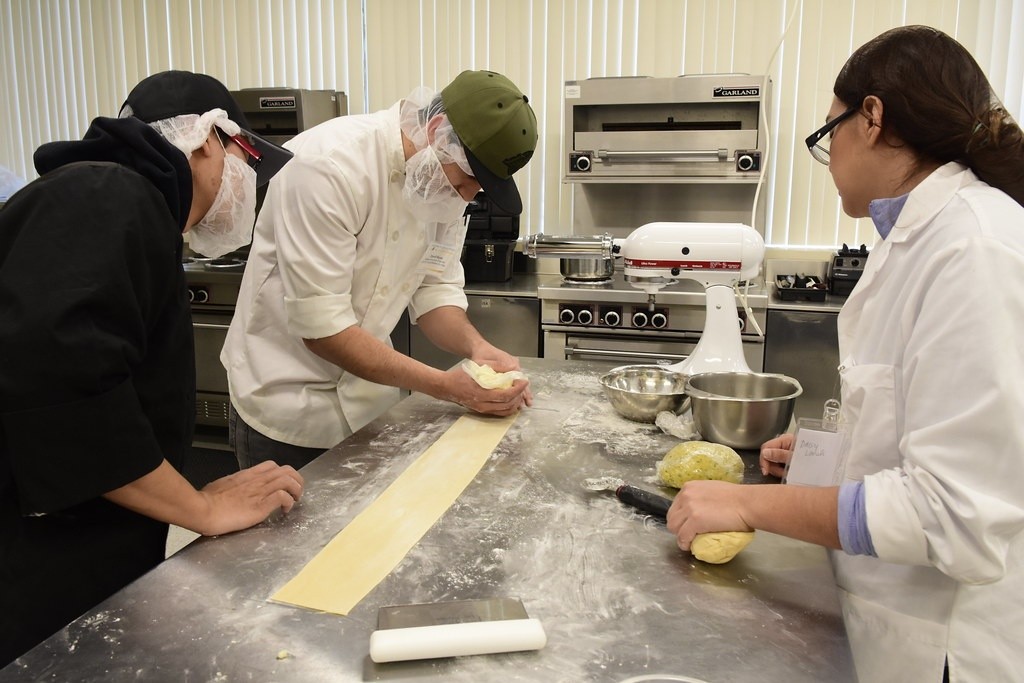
<box><xmin>581</xmin><ymin>478</ymin><xmax>673</xmax><ymax>518</ymax></box>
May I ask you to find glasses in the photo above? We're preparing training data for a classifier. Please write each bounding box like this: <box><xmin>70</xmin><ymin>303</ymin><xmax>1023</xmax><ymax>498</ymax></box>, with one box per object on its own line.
<box><xmin>230</xmin><ymin>134</ymin><xmax>264</xmax><ymax>172</ymax></box>
<box><xmin>805</xmin><ymin>94</ymin><xmax>889</xmax><ymax>166</ymax></box>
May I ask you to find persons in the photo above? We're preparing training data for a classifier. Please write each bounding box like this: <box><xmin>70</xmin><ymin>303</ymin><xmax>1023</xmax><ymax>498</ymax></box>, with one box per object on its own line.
<box><xmin>0</xmin><ymin>70</ymin><xmax>305</xmax><ymax>671</ymax></box>
<box><xmin>664</xmin><ymin>24</ymin><xmax>1024</xmax><ymax>683</ymax></box>
<box><xmin>218</xmin><ymin>66</ymin><xmax>540</xmax><ymax>474</ymax></box>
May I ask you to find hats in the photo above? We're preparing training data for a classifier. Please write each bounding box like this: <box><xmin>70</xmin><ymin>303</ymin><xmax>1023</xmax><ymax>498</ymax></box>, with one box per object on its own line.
<box><xmin>118</xmin><ymin>70</ymin><xmax>294</xmax><ymax>187</ymax></box>
<box><xmin>441</xmin><ymin>70</ymin><xmax>539</xmax><ymax>215</ymax></box>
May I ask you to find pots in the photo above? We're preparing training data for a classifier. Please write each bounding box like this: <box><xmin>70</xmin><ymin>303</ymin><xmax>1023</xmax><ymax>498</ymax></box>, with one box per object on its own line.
<box><xmin>560</xmin><ymin>257</ymin><xmax>615</xmax><ymax>279</ymax></box>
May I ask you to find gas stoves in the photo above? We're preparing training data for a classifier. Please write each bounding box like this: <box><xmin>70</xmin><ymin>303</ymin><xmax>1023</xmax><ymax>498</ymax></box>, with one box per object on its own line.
<box><xmin>182</xmin><ymin>254</ymin><xmax>247</xmax><ymax>305</ymax></box>
<box><xmin>538</xmin><ymin>265</ymin><xmax>768</xmax><ymax>335</ymax></box>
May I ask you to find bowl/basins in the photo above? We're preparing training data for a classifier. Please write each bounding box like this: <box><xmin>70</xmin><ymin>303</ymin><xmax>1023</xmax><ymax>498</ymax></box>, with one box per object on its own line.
<box><xmin>599</xmin><ymin>370</ymin><xmax>690</xmax><ymax>424</ymax></box>
<box><xmin>684</xmin><ymin>371</ymin><xmax>803</xmax><ymax>450</ymax></box>
<box><xmin>609</xmin><ymin>365</ymin><xmax>672</xmax><ymax>371</ymax></box>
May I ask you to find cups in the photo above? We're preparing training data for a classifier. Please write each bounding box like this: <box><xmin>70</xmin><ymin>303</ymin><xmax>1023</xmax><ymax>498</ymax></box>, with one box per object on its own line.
<box><xmin>822</xmin><ymin>399</ymin><xmax>840</xmax><ymax>431</ymax></box>
